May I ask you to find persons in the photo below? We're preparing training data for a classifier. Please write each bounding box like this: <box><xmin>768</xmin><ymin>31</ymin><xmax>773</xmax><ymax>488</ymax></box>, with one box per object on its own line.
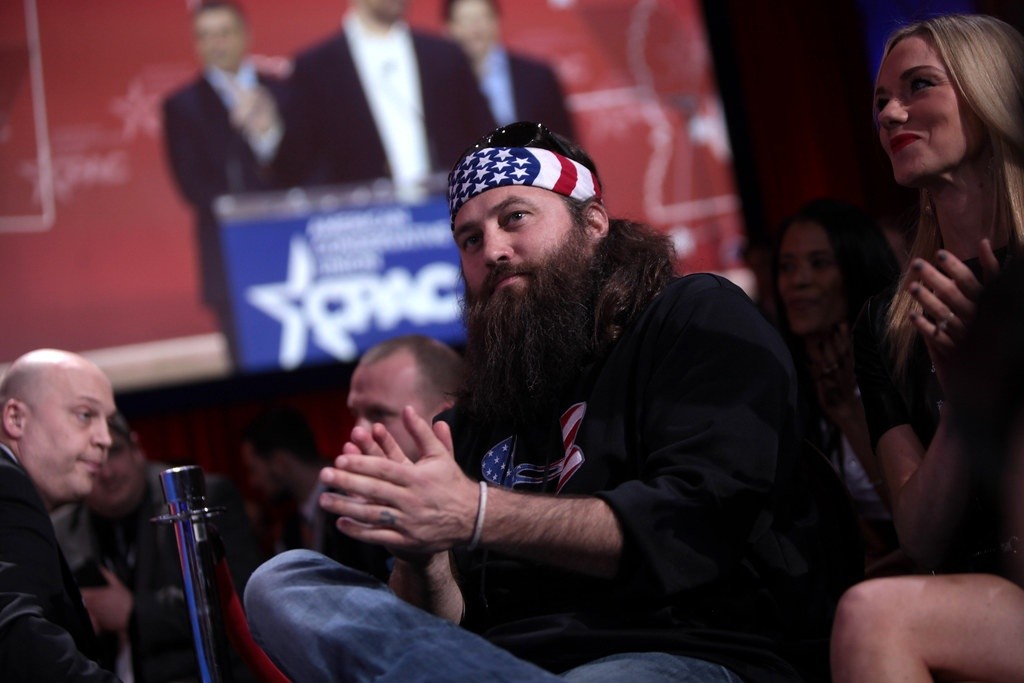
<box><xmin>157</xmin><ymin>1</ymin><xmax>578</xmax><ymax>373</ymax></box>
<box><xmin>307</xmin><ymin>331</ymin><xmax>479</xmax><ymax>584</ymax></box>
<box><xmin>766</xmin><ymin>193</ymin><xmax>874</xmax><ymax>409</ymax></box>
<box><xmin>242</xmin><ymin>115</ymin><xmax>803</xmax><ymax>683</ymax></box>
<box><xmin>825</xmin><ymin>10</ymin><xmax>1024</xmax><ymax>683</ymax></box>
<box><xmin>226</xmin><ymin>392</ymin><xmax>361</xmax><ymax>570</ymax></box>
<box><xmin>460</xmin><ymin>481</ymin><xmax>489</xmax><ymax>552</ymax></box>
<box><xmin>0</xmin><ymin>348</ymin><xmax>245</xmax><ymax>683</ymax></box>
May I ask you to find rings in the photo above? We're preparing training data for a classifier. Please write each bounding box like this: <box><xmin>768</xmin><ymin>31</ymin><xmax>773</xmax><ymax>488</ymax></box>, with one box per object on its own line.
<box><xmin>935</xmin><ymin>312</ymin><xmax>955</xmax><ymax>332</ymax></box>
